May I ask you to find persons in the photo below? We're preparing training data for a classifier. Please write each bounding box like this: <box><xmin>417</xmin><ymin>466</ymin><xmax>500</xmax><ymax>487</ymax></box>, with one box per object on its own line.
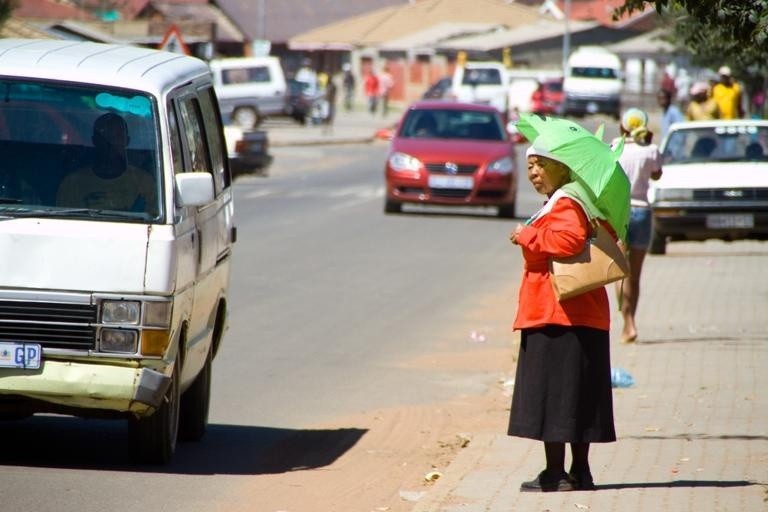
<box><xmin>609</xmin><ymin>107</ymin><xmax>662</xmax><ymax>345</ymax></box>
<box><xmin>656</xmin><ymin>59</ymin><xmax>766</xmax><ymax>162</ymax></box>
<box><xmin>56</xmin><ymin>113</ymin><xmax>158</xmax><ymax>214</ymax></box>
<box><xmin>294</xmin><ymin>56</ymin><xmax>394</xmax><ymax>124</ymax></box>
<box><xmin>507</xmin><ymin>146</ymin><xmax>617</xmax><ymax>494</ymax></box>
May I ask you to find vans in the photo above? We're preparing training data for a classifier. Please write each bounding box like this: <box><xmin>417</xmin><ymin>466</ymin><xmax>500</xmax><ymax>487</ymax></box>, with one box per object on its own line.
<box><xmin>200</xmin><ymin>56</ymin><xmax>303</xmax><ymax>131</ymax></box>
<box><xmin>449</xmin><ymin>59</ymin><xmax>511</xmax><ymax>122</ymax></box>
<box><xmin>560</xmin><ymin>46</ymin><xmax>627</xmax><ymax>122</ymax></box>
<box><xmin>0</xmin><ymin>38</ymin><xmax>237</xmax><ymax>468</ymax></box>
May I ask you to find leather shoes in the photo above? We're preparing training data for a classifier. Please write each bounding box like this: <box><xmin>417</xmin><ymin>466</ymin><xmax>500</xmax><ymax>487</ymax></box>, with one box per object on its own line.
<box><xmin>520</xmin><ymin>470</ymin><xmax>569</xmax><ymax>492</ymax></box>
<box><xmin>558</xmin><ymin>469</ymin><xmax>593</xmax><ymax>491</ymax></box>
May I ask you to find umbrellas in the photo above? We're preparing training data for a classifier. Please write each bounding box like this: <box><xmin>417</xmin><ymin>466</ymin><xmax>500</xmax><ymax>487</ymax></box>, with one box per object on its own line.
<box><xmin>511</xmin><ymin>111</ymin><xmax>632</xmax><ymax>244</ymax></box>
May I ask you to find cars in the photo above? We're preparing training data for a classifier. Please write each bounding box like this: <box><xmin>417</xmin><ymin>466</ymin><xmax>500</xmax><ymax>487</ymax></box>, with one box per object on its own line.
<box><xmin>646</xmin><ymin>118</ymin><xmax>768</xmax><ymax>255</ymax></box>
<box><xmin>422</xmin><ymin>70</ymin><xmax>564</xmax><ymax>120</ymax></box>
<box><xmin>373</xmin><ymin>101</ymin><xmax>529</xmax><ymax>219</ymax></box>
<box><xmin>219</xmin><ymin>121</ymin><xmax>274</xmax><ymax>185</ymax></box>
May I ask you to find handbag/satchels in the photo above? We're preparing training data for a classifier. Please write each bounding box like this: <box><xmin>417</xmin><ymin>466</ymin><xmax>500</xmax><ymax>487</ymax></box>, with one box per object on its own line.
<box><xmin>547</xmin><ymin>191</ymin><xmax>631</xmax><ymax>303</ymax></box>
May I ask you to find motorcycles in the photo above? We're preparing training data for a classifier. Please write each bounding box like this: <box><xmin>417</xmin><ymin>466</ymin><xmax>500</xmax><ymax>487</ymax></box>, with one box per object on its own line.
<box><xmin>305</xmin><ymin>82</ymin><xmax>337</xmax><ymax>128</ymax></box>
